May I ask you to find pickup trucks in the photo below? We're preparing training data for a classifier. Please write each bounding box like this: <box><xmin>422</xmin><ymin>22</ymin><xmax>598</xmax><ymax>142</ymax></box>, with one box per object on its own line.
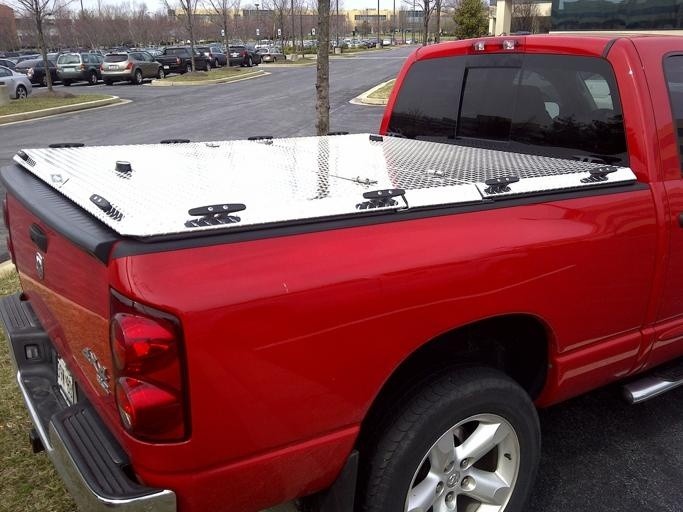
<box><xmin>0</xmin><ymin>24</ymin><xmax>681</xmax><ymax>512</ymax></box>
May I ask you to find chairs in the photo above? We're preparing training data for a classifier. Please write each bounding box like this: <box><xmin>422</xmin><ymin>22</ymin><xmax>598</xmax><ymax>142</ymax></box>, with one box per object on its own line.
<box><xmin>474</xmin><ymin>81</ymin><xmax>621</xmax><ymax>154</ymax></box>
